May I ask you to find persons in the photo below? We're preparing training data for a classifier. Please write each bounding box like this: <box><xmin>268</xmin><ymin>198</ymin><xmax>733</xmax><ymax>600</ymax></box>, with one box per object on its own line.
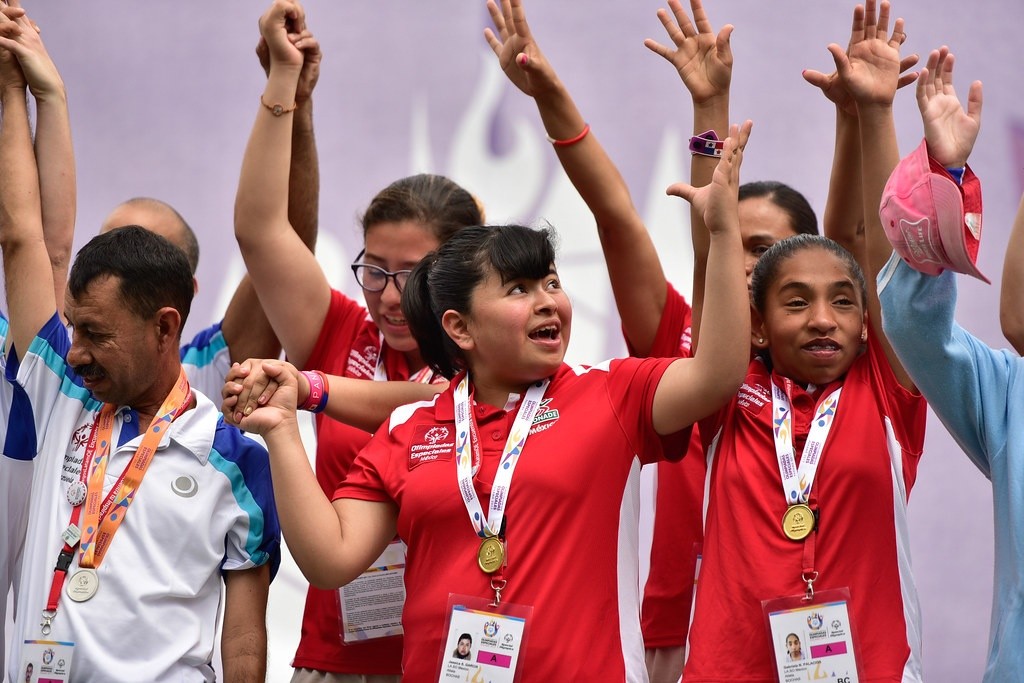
<box><xmin>784</xmin><ymin>633</ymin><xmax>806</xmax><ymax>662</ymax></box>
<box><xmin>241</xmin><ymin>0</ymin><xmax>453</xmax><ymax>683</ymax></box>
<box><xmin>0</xmin><ymin>0</ymin><xmax>317</xmax><ymax>683</ymax></box>
<box><xmin>483</xmin><ymin>0</ymin><xmax>917</xmax><ymax>683</ymax></box>
<box><xmin>223</xmin><ymin>121</ymin><xmax>754</xmax><ymax>683</ymax></box>
<box><xmin>646</xmin><ymin>0</ymin><xmax>927</xmax><ymax>683</ymax></box>
<box><xmin>24</xmin><ymin>662</ymin><xmax>34</xmax><ymax>683</ymax></box>
<box><xmin>874</xmin><ymin>47</ymin><xmax>1024</xmax><ymax>683</ymax></box>
<box><xmin>451</xmin><ymin>632</ymin><xmax>472</xmax><ymax>661</ymax></box>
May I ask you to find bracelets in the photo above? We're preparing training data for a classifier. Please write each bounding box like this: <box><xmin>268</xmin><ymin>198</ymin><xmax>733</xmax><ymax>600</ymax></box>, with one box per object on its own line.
<box><xmin>260</xmin><ymin>94</ymin><xmax>298</xmax><ymax>118</ymax></box>
<box><xmin>689</xmin><ymin>129</ymin><xmax>724</xmax><ymax>157</ymax></box>
<box><xmin>947</xmin><ymin>166</ymin><xmax>967</xmax><ymax>184</ymax></box>
<box><xmin>314</xmin><ymin>370</ymin><xmax>329</xmax><ymax>414</ymax></box>
<box><xmin>544</xmin><ymin>123</ymin><xmax>590</xmax><ymax>145</ymax></box>
<box><xmin>301</xmin><ymin>370</ymin><xmax>322</xmax><ymax>411</ymax></box>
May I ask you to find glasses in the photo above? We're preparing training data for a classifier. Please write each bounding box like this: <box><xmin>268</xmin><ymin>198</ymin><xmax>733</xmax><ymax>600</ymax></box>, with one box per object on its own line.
<box><xmin>352</xmin><ymin>248</ymin><xmax>413</xmax><ymax>296</ymax></box>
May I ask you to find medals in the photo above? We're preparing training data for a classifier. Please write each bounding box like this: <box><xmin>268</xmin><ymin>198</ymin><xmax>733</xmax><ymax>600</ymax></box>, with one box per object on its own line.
<box><xmin>477</xmin><ymin>538</ymin><xmax>503</xmax><ymax>572</ymax></box>
<box><xmin>66</xmin><ymin>568</ymin><xmax>100</xmax><ymax>603</ymax></box>
<box><xmin>781</xmin><ymin>505</ymin><xmax>815</xmax><ymax>540</ymax></box>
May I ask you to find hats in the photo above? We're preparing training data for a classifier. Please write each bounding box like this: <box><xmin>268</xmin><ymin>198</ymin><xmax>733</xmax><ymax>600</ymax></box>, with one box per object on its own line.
<box><xmin>879</xmin><ymin>135</ymin><xmax>992</xmax><ymax>286</ymax></box>
